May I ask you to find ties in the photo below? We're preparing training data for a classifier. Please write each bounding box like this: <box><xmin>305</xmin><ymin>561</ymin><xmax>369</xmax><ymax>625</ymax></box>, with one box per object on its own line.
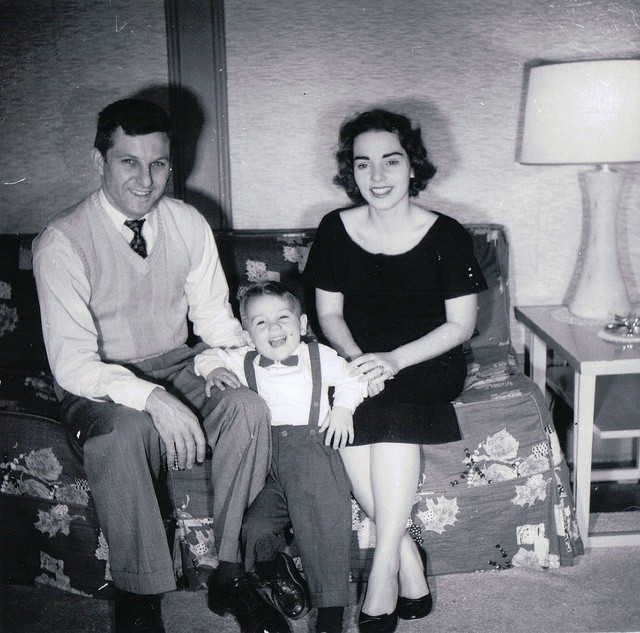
<box><xmin>125</xmin><ymin>217</ymin><xmax>146</xmax><ymax>259</ymax></box>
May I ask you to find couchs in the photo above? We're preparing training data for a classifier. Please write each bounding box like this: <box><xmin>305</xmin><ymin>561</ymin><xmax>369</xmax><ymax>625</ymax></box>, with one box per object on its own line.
<box><xmin>1</xmin><ymin>222</ymin><xmax>583</xmax><ymax>599</ymax></box>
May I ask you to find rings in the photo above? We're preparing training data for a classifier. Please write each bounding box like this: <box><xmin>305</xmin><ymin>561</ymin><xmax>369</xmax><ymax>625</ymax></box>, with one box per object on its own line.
<box><xmin>379</xmin><ymin>365</ymin><xmax>386</xmax><ymax>374</ymax></box>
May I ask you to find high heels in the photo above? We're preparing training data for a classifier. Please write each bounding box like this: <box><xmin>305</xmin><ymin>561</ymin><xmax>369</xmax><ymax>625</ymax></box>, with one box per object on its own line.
<box><xmin>399</xmin><ymin>540</ymin><xmax>431</xmax><ymax>619</ymax></box>
<box><xmin>358</xmin><ymin>571</ymin><xmax>398</xmax><ymax>631</ymax></box>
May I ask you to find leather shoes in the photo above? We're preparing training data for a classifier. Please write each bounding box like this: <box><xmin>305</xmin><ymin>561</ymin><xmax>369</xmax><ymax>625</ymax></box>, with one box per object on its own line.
<box><xmin>267</xmin><ymin>549</ymin><xmax>309</xmax><ymax>619</ymax></box>
<box><xmin>205</xmin><ymin>569</ymin><xmax>291</xmax><ymax>633</ymax></box>
<box><xmin>118</xmin><ymin>594</ymin><xmax>166</xmax><ymax>632</ymax></box>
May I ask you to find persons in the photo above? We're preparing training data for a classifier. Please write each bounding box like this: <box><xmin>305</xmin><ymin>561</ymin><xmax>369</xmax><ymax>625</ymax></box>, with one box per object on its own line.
<box><xmin>30</xmin><ymin>101</ymin><xmax>290</xmax><ymax>633</ymax></box>
<box><xmin>302</xmin><ymin>108</ymin><xmax>488</xmax><ymax>632</ymax></box>
<box><xmin>194</xmin><ymin>281</ymin><xmax>371</xmax><ymax>632</ymax></box>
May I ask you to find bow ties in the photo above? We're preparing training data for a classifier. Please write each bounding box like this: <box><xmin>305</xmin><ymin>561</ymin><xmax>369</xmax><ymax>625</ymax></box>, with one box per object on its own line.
<box><xmin>258</xmin><ymin>355</ymin><xmax>299</xmax><ymax>366</ymax></box>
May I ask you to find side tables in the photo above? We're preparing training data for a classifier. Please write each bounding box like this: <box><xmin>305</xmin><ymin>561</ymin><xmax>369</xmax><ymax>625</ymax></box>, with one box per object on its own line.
<box><xmin>513</xmin><ymin>306</ymin><xmax>640</xmax><ymax>550</ymax></box>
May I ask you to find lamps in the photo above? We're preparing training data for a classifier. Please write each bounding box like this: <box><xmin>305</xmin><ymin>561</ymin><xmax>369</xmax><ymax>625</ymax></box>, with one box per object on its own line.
<box><xmin>519</xmin><ymin>59</ymin><xmax>640</xmax><ymax>344</ymax></box>
